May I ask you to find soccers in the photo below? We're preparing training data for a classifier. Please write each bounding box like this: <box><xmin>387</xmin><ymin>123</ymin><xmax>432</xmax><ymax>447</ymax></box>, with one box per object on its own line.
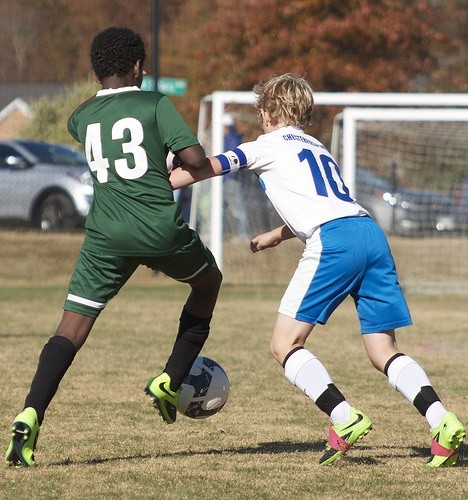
<box><xmin>173</xmin><ymin>355</ymin><xmax>230</xmax><ymax>420</ymax></box>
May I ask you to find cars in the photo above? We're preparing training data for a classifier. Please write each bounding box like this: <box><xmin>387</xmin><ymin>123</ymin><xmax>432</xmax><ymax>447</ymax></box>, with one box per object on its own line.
<box><xmin>356</xmin><ymin>170</ymin><xmax>468</xmax><ymax>236</ymax></box>
<box><xmin>0</xmin><ymin>139</ymin><xmax>94</xmax><ymax>231</ymax></box>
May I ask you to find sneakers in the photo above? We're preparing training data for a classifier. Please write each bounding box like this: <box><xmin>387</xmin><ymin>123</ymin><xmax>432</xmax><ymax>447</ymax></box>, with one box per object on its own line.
<box><xmin>425</xmin><ymin>411</ymin><xmax>465</xmax><ymax>469</ymax></box>
<box><xmin>143</xmin><ymin>370</ymin><xmax>184</xmax><ymax>424</ymax></box>
<box><xmin>5</xmin><ymin>406</ymin><xmax>40</xmax><ymax>470</ymax></box>
<box><xmin>316</xmin><ymin>408</ymin><xmax>374</xmax><ymax>466</ymax></box>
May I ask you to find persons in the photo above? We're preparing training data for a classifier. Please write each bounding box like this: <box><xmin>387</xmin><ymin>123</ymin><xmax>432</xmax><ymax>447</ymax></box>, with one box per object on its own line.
<box><xmin>5</xmin><ymin>26</ymin><xmax>223</xmax><ymax>470</ymax></box>
<box><xmin>219</xmin><ymin>114</ymin><xmax>248</xmax><ymax>234</ymax></box>
<box><xmin>377</xmin><ymin>151</ymin><xmax>405</xmax><ymax>193</ymax></box>
<box><xmin>165</xmin><ymin>72</ymin><xmax>467</xmax><ymax>471</ymax></box>
<box><xmin>236</xmin><ymin>117</ymin><xmax>270</xmax><ymax>233</ymax></box>
